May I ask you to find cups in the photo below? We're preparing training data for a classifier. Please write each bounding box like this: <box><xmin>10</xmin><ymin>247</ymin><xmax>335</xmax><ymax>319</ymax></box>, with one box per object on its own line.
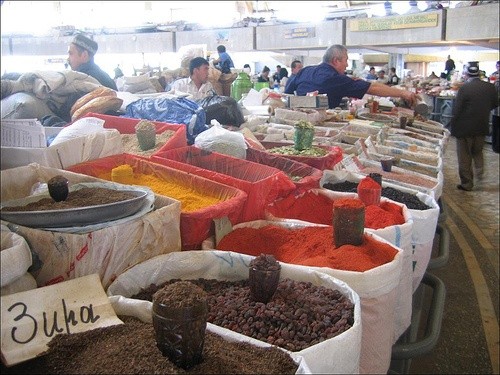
<box><xmin>357</xmin><ymin>188</ymin><xmax>381</xmax><ymax>207</ymax></box>
<box><xmin>249</xmin><ymin>267</ymin><xmax>281</xmax><ymax>302</ymax></box>
<box><xmin>400</xmin><ymin>117</ymin><xmax>407</xmax><ymax>129</ymax></box>
<box><xmin>136</xmin><ymin>127</ymin><xmax>156</xmax><ymax>151</ymax></box>
<box><xmin>333</xmin><ymin>199</ymin><xmax>365</xmax><ymax>249</ymax></box>
<box><xmin>407</xmin><ymin>118</ymin><xmax>414</xmax><ymax>126</ymax></box>
<box><xmin>48</xmin><ymin>180</ymin><xmax>69</xmax><ymax>202</ymax></box>
<box><xmin>294</xmin><ymin>127</ymin><xmax>314</xmax><ymax>151</ymax></box>
<box><xmin>152</xmin><ymin>299</ymin><xmax>208</xmax><ymax>370</ymax></box>
<box><xmin>381</xmin><ymin>159</ymin><xmax>392</xmax><ymax>172</ymax></box>
<box><xmin>112</xmin><ymin>168</ymin><xmax>134</xmax><ymax>186</ymax></box>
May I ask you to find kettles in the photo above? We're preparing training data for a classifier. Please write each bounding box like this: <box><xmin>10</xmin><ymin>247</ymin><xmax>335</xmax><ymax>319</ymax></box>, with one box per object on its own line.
<box><xmin>230</xmin><ymin>73</ymin><xmax>252</xmax><ymax>102</ymax></box>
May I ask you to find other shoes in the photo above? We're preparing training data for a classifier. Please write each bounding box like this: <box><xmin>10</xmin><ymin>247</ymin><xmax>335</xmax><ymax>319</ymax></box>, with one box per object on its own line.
<box><xmin>457</xmin><ymin>185</ymin><xmax>465</xmax><ymax>190</ymax></box>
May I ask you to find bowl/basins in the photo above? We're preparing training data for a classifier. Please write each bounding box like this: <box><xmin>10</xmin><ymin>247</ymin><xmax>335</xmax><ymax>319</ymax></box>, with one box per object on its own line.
<box><xmin>357</xmin><ymin>113</ymin><xmax>395</xmax><ymax>123</ymax></box>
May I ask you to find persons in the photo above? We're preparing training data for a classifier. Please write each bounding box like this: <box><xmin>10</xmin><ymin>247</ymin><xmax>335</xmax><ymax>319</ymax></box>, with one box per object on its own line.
<box><xmin>213</xmin><ymin>44</ymin><xmax>235</xmax><ymax>73</ymax></box>
<box><xmin>445</xmin><ymin>54</ymin><xmax>500</xmax><ymax>191</ymax></box>
<box><xmin>168</xmin><ymin>57</ymin><xmax>216</xmax><ymax>100</ymax></box>
<box><xmin>285</xmin><ymin>45</ymin><xmax>422</xmax><ymax>109</ymax></box>
<box><xmin>65</xmin><ymin>33</ymin><xmax>120</xmax><ymax>91</ymax></box>
<box><xmin>243</xmin><ymin>60</ymin><xmax>303</xmax><ymax>92</ymax></box>
<box><xmin>367</xmin><ymin>67</ymin><xmax>400</xmax><ymax>85</ymax></box>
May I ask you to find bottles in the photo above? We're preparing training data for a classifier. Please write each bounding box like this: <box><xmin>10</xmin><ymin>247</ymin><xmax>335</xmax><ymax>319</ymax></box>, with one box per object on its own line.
<box><xmin>273</xmin><ymin>85</ymin><xmax>280</xmax><ymax>93</ymax></box>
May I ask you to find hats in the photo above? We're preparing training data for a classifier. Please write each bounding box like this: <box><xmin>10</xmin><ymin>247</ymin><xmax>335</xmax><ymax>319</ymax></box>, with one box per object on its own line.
<box><xmin>72</xmin><ymin>34</ymin><xmax>98</xmax><ymax>54</ymax></box>
<box><xmin>465</xmin><ymin>62</ymin><xmax>480</xmax><ymax>76</ymax></box>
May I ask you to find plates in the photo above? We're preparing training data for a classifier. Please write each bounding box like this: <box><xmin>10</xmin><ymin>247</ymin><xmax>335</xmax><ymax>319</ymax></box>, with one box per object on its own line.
<box><xmin>0</xmin><ymin>190</ymin><xmax>148</xmax><ymax>228</ymax></box>
